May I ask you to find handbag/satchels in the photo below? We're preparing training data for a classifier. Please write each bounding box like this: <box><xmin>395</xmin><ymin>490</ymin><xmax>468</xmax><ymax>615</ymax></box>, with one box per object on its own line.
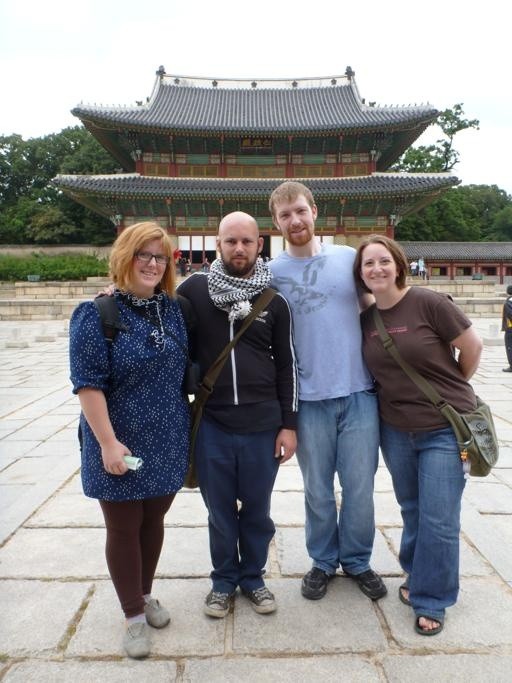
<box><xmin>440</xmin><ymin>394</ymin><xmax>499</xmax><ymax>476</ymax></box>
<box><xmin>183</xmin><ymin>401</ymin><xmax>203</xmax><ymax>488</ymax></box>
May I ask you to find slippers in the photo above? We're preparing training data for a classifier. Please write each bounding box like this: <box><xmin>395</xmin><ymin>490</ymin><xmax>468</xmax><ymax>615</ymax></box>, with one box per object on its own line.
<box><xmin>400</xmin><ymin>577</ymin><xmax>444</xmax><ymax>635</ymax></box>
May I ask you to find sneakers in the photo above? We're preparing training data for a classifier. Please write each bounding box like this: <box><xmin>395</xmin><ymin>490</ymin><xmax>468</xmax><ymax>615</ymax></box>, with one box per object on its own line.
<box><xmin>127</xmin><ymin>622</ymin><xmax>149</xmax><ymax>657</ymax></box>
<box><xmin>301</xmin><ymin>567</ymin><xmax>329</xmax><ymax>600</ymax></box>
<box><xmin>143</xmin><ymin>598</ymin><xmax>170</xmax><ymax>628</ymax></box>
<box><xmin>248</xmin><ymin>586</ymin><xmax>276</xmax><ymax>613</ymax></box>
<box><xmin>353</xmin><ymin>570</ymin><xmax>386</xmax><ymax>600</ymax></box>
<box><xmin>204</xmin><ymin>590</ymin><xmax>235</xmax><ymax>618</ymax></box>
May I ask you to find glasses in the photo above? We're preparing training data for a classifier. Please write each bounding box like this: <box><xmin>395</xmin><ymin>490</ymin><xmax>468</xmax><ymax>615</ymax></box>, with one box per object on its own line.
<box><xmin>135</xmin><ymin>252</ymin><xmax>169</xmax><ymax>264</ymax></box>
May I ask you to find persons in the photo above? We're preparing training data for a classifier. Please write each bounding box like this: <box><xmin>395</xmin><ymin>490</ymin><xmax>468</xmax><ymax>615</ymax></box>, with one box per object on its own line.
<box><xmin>500</xmin><ymin>285</ymin><xmax>512</xmax><ymax>372</ymax></box>
<box><xmin>410</xmin><ymin>260</ymin><xmax>418</xmax><ymax>276</ymax></box>
<box><xmin>175</xmin><ymin>210</ymin><xmax>298</xmax><ymax>618</ymax></box>
<box><xmin>418</xmin><ymin>255</ymin><xmax>424</xmax><ymax>276</ymax></box>
<box><xmin>266</xmin><ymin>181</ymin><xmax>387</xmax><ymax>600</ymax></box>
<box><xmin>353</xmin><ymin>235</ymin><xmax>483</xmax><ymax>636</ymax></box>
<box><xmin>186</xmin><ymin>258</ymin><xmax>191</xmax><ymax>272</ymax></box>
<box><xmin>202</xmin><ymin>257</ymin><xmax>210</xmax><ymax>272</ymax></box>
<box><xmin>180</xmin><ymin>258</ymin><xmax>186</xmax><ymax>276</ymax></box>
<box><xmin>257</xmin><ymin>253</ymin><xmax>264</xmax><ymax>263</ymax></box>
<box><xmin>69</xmin><ymin>221</ymin><xmax>190</xmax><ymax>658</ymax></box>
